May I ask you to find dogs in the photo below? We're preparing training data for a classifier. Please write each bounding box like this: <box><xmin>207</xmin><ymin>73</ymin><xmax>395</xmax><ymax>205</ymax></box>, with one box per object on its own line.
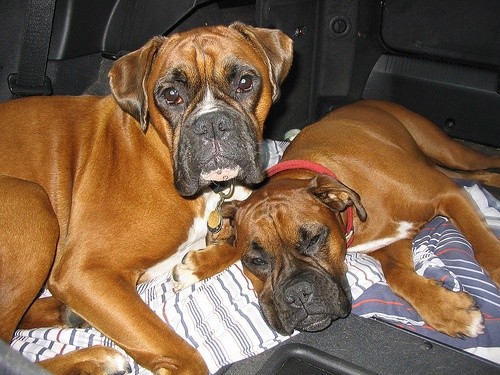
<box><xmin>185</xmin><ymin>98</ymin><xmax>499</xmax><ymax>338</ymax></box>
<box><xmin>0</xmin><ymin>20</ymin><xmax>295</xmax><ymax>374</ymax></box>
